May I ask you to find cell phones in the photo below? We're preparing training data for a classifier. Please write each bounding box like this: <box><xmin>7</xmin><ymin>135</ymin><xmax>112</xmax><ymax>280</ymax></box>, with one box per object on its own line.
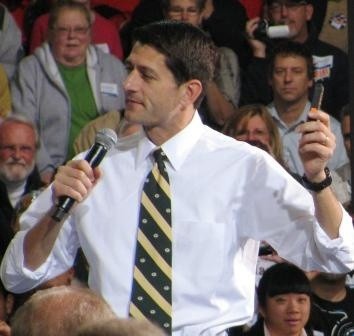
<box><xmin>303</xmin><ymin>84</ymin><xmax>324</xmax><ymax>142</ymax></box>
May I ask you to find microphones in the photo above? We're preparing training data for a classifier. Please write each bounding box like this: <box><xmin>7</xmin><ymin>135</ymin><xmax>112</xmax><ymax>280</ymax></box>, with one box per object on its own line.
<box><xmin>52</xmin><ymin>126</ymin><xmax>119</xmax><ymax>224</ymax></box>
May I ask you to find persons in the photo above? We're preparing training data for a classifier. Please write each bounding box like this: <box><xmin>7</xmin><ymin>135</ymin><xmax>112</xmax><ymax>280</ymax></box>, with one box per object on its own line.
<box><xmin>339</xmin><ymin>103</ymin><xmax>353</xmax><ymax>166</ymax></box>
<box><xmin>161</xmin><ymin>0</ymin><xmax>241</xmax><ymax>131</ymax></box>
<box><xmin>0</xmin><ymin>111</ymin><xmax>74</xmax><ymax>328</ymax></box>
<box><xmin>0</xmin><ymin>3</ymin><xmax>24</xmax><ymax>80</ymax></box>
<box><xmin>40</xmin><ymin>267</ymin><xmax>75</xmax><ymax>289</ymax></box>
<box><xmin>72</xmin><ymin>107</ymin><xmax>143</xmax><ymax>155</ymax></box>
<box><xmin>221</xmin><ymin>103</ymin><xmax>281</xmax><ymax>162</ymax></box>
<box><xmin>242</xmin><ymin>262</ymin><xmax>328</xmax><ymax>336</ymax></box>
<box><xmin>9</xmin><ymin>284</ymin><xmax>117</xmax><ymax>336</ymax></box>
<box><xmin>8</xmin><ymin>1</ymin><xmax>129</xmax><ymax>185</ymax></box>
<box><xmin>0</xmin><ymin>278</ymin><xmax>13</xmax><ymax>336</ymax></box>
<box><xmin>0</xmin><ymin>19</ymin><xmax>354</xmax><ymax>336</ymax></box>
<box><xmin>0</xmin><ymin>63</ymin><xmax>11</xmax><ymax>117</ymax></box>
<box><xmin>264</xmin><ymin>38</ymin><xmax>352</xmax><ymax>209</ymax></box>
<box><xmin>242</xmin><ymin>0</ymin><xmax>353</xmax><ymax>122</ymax></box>
<box><xmin>29</xmin><ymin>0</ymin><xmax>123</xmax><ymax>62</ymax></box>
<box><xmin>65</xmin><ymin>315</ymin><xmax>169</xmax><ymax>336</ymax></box>
<box><xmin>303</xmin><ymin>273</ymin><xmax>353</xmax><ymax>336</ymax></box>
<box><xmin>309</xmin><ymin>0</ymin><xmax>354</xmax><ymax>56</ymax></box>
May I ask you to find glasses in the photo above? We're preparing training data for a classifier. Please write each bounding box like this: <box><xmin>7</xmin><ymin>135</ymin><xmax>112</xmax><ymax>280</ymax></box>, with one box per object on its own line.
<box><xmin>268</xmin><ymin>2</ymin><xmax>309</xmax><ymax>12</ymax></box>
<box><xmin>49</xmin><ymin>24</ymin><xmax>92</xmax><ymax>34</ymax></box>
<box><xmin>168</xmin><ymin>6</ymin><xmax>201</xmax><ymax>17</ymax></box>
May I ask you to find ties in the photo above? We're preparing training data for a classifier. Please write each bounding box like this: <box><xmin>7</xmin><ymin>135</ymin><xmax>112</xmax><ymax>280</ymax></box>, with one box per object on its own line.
<box><xmin>129</xmin><ymin>149</ymin><xmax>172</xmax><ymax>335</ymax></box>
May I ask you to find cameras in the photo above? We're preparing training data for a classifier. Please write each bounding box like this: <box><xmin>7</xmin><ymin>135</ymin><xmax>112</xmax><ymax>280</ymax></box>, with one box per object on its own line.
<box><xmin>256</xmin><ymin>18</ymin><xmax>291</xmax><ymax>44</ymax></box>
